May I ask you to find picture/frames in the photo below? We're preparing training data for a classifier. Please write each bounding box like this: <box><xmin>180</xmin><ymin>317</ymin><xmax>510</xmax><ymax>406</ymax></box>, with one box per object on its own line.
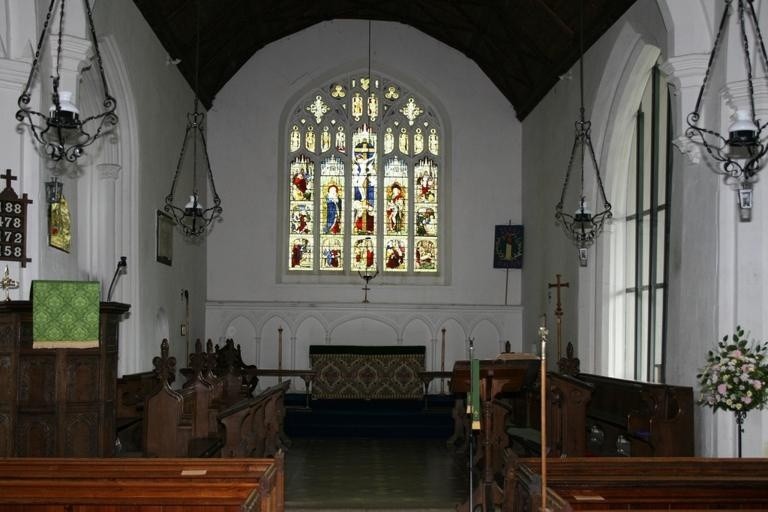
<box><xmin>156</xmin><ymin>210</ymin><xmax>172</xmax><ymax>267</ymax></box>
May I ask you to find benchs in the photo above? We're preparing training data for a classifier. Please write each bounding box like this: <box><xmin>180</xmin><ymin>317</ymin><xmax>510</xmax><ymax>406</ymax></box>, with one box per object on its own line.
<box><xmin>1</xmin><ymin>450</ymin><xmax>288</xmax><ymax>510</ymax></box>
<box><xmin>444</xmin><ymin>325</ymin><xmax>767</xmax><ymax>511</ymax></box>
<box><xmin>110</xmin><ymin>337</ymin><xmax>291</xmax><ymax>459</ymax></box>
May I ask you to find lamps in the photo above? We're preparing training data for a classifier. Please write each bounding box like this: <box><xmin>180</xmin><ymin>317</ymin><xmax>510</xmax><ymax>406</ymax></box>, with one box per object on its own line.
<box><xmin>161</xmin><ymin>0</ymin><xmax>224</xmax><ymax>235</ymax></box>
<box><xmin>555</xmin><ymin>1</ymin><xmax>614</xmax><ymax>270</ymax></box>
<box><xmin>671</xmin><ymin>1</ymin><xmax>767</xmax><ymax>223</ymax></box>
<box><xmin>13</xmin><ymin>2</ymin><xmax>121</xmax><ymax>168</ymax></box>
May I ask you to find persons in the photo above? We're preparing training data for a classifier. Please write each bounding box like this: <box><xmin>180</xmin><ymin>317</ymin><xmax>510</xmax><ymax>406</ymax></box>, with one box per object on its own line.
<box><xmin>292</xmin><ymin>167</ymin><xmax>437</xmax><ymax>271</ymax></box>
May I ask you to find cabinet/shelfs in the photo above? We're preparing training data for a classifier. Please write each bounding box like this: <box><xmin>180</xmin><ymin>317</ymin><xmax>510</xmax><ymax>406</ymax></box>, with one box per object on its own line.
<box><xmin>1</xmin><ymin>299</ymin><xmax>131</xmax><ymax>458</ymax></box>
